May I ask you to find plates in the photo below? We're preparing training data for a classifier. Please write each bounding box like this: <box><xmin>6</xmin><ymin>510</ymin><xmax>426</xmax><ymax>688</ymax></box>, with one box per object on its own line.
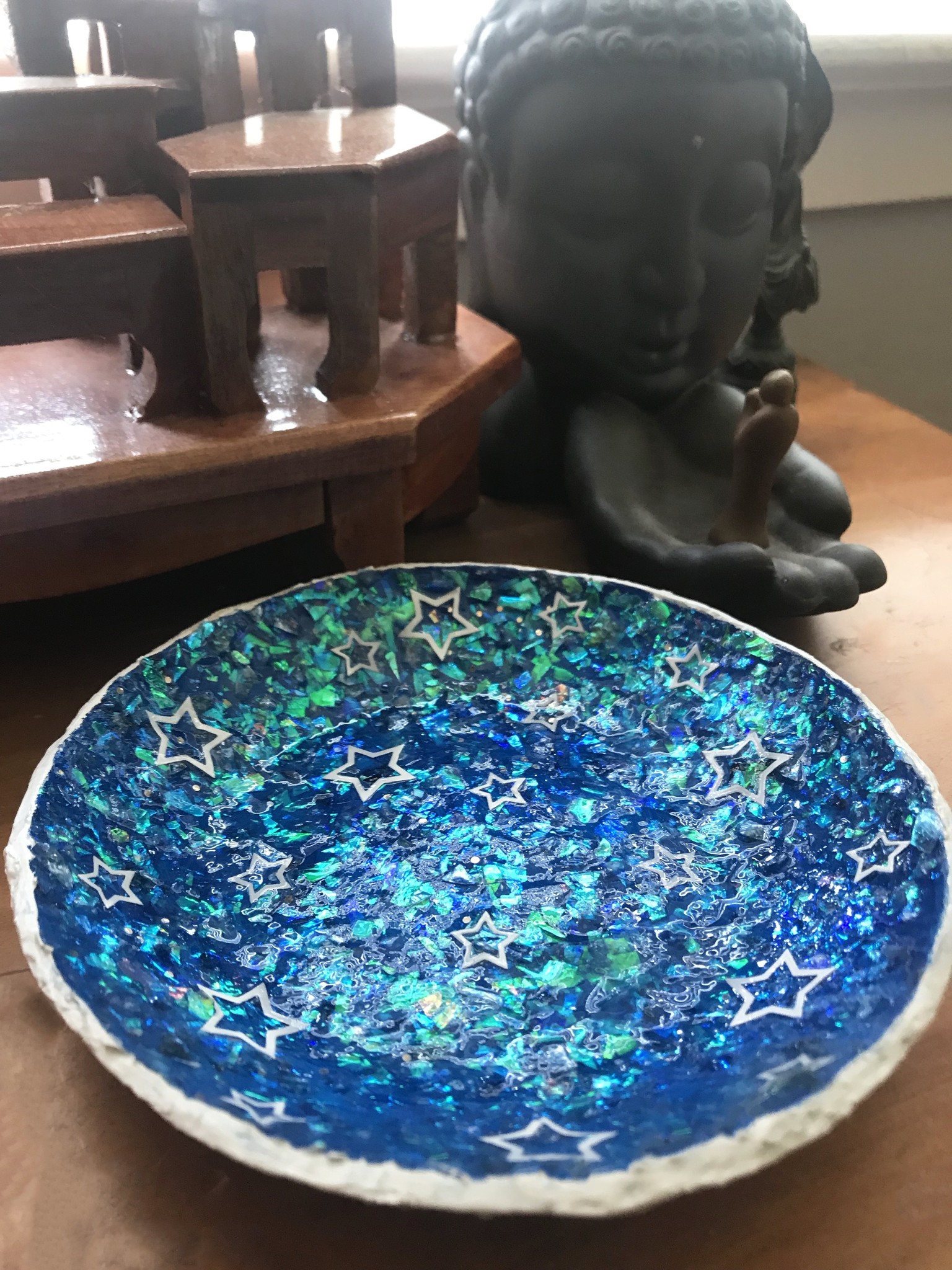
<box><xmin>0</xmin><ymin>561</ymin><xmax>951</xmax><ymax>1220</ymax></box>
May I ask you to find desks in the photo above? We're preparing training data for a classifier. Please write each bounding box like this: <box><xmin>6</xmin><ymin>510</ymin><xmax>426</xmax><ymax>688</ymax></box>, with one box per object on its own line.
<box><xmin>0</xmin><ymin>336</ymin><xmax>952</xmax><ymax>1270</ymax></box>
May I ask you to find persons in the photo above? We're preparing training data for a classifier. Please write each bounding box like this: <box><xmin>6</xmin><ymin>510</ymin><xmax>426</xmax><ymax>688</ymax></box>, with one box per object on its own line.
<box><xmin>450</xmin><ymin>0</ymin><xmax>889</xmax><ymax>628</ymax></box>
